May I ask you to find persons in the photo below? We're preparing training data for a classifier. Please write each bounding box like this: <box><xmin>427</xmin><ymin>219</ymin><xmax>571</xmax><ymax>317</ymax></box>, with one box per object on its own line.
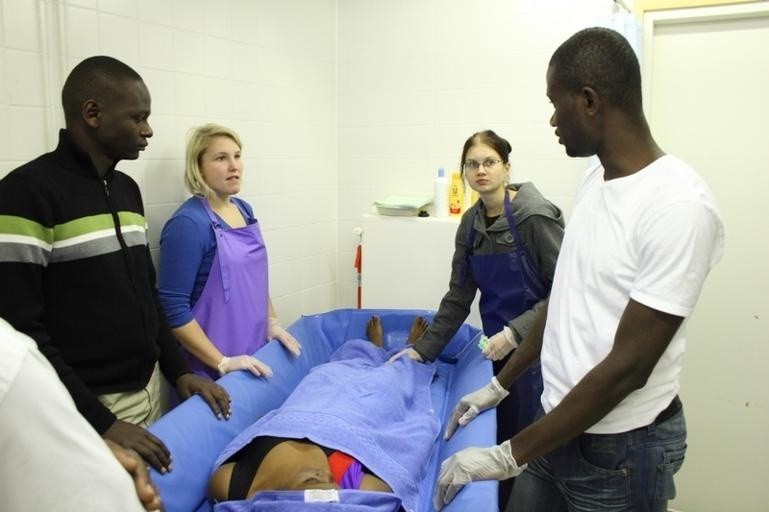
<box><xmin>434</xmin><ymin>26</ymin><xmax>726</xmax><ymax>512</ymax></box>
<box><xmin>206</xmin><ymin>313</ymin><xmax>431</xmax><ymax>502</ymax></box>
<box><xmin>0</xmin><ymin>315</ymin><xmax>166</xmax><ymax>512</ymax></box>
<box><xmin>385</xmin><ymin>127</ymin><xmax>565</xmax><ymax>511</ymax></box>
<box><xmin>0</xmin><ymin>55</ymin><xmax>233</xmax><ymax>475</ymax></box>
<box><xmin>155</xmin><ymin>120</ymin><xmax>301</xmax><ymax>381</ymax></box>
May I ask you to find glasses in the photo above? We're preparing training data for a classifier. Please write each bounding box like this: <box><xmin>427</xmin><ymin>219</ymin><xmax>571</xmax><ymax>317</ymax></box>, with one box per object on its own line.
<box><xmin>465</xmin><ymin>158</ymin><xmax>503</xmax><ymax>168</ymax></box>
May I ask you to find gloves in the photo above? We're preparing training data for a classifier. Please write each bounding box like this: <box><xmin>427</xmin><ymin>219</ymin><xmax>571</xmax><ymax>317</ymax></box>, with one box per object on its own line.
<box><xmin>217</xmin><ymin>354</ymin><xmax>273</xmax><ymax>379</ymax></box>
<box><xmin>433</xmin><ymin>440</ymin><xmax>529</xmax><ymax>512</ymax></box>
<box><xmin>267</xmin><ymin>318</ymin><xmax>302</xmax><ymax>360</ymax></box>
<box><xmin>443</xmin><ymin>376</ymin><xmax>510</xmax><ymax>441</ymax></box>
<box><xmin>386</xmin><ymin>348</ymin><xmax>424</xmax><ymax>369</ymax></box>
<box><xmin>481</xmin><ymin>325</ymin><xmax>518</xmax><ymax>362</ymax></box>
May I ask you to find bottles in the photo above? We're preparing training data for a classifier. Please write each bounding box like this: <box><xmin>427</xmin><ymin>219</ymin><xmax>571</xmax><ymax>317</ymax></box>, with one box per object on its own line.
<box><xmin>432</xmin><ymin>168</ymin><xmax>449</xmax><ymax>219</ymax></box>
<box><xmin>449</xmin><ymin>172</ymin><xmax>465</xmax><ymax>215</ymax></box>
